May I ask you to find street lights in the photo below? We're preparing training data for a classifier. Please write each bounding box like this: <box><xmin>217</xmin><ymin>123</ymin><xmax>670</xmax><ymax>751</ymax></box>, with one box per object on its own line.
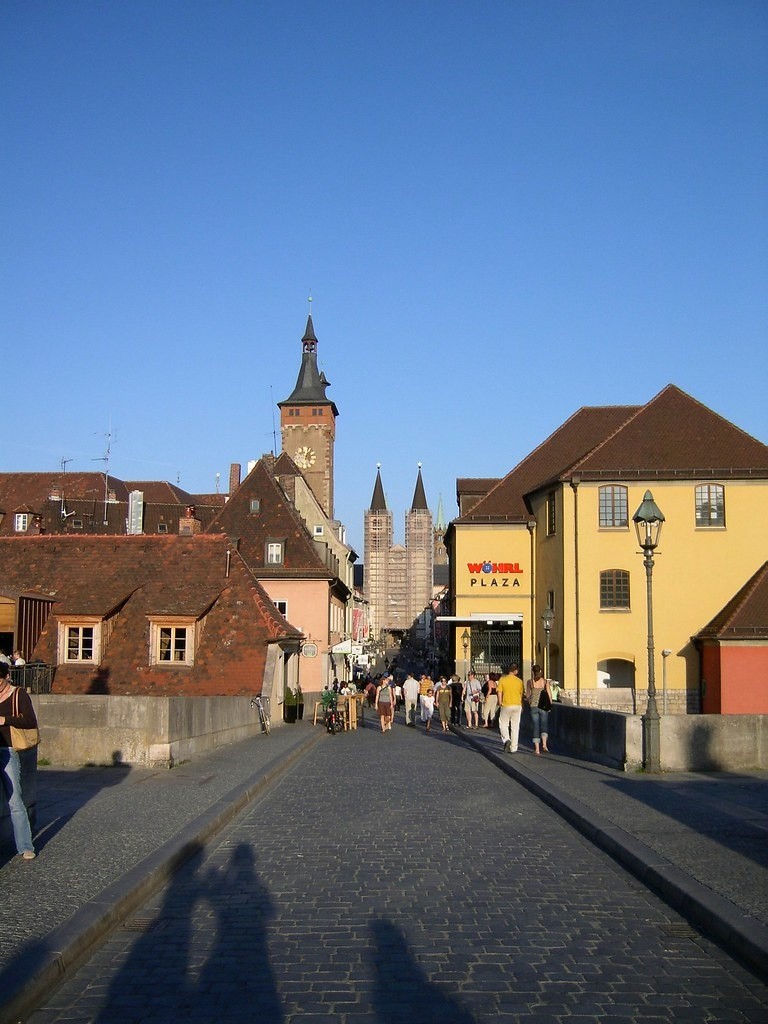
<box><xmin>540</xmin><ymin>609</ymin><xmax>555</xmax><ymax>680</ymax></box>
<box><xmin>631</xmin><ymin>490</ymin><xmax>666</xmax><ymax>773</ymax></box>
<box><xmin>460</xmin><ymin>629</ymin><xmax>471</xmax><ymax>682</ymax></box>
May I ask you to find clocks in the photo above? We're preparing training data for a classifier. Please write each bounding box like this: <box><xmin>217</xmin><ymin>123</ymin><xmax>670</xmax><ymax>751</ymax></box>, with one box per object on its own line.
<box><xmin>294</xmin><ymin>446</ymin><xmax>316</xmax><ymax>469</ymax></box>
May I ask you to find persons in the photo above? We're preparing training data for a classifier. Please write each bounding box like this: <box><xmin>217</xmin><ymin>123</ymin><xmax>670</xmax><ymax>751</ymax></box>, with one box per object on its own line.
<box><xmin>323</xmin><ymin>686</ymin><xmax>328</xmax><ymax>691</ymax></box>
<box><xmin>375</xmin><ymin>652</ymin><xmax>439</xmax><ymax>712</ymax></box>
<box><xmin>332</xmin><ymin>672</ymin><xmax>376</xmax><ymax>707</ymax></box>
<box><xmin>461</xmin><ymin>671</ymin><xmax>482</xmax><ymax>729</ymax></box>
<box><xmin>419</xmin><ymin>675</ymin><xmax>432</xmax><ymax>724</ymax></box>
<box><xmin>526</xmin><ymin>665</ymin><xmax>552</xmax><ymax>754</ymax></box>
<box><xmin>0</xmin><ymin>662</ymin><xmax>40</xmax><ymax>859</ymax></box>
<box><xmin>14</xmin><ymin>651</ymin><xmax>25</xmax><ymax>665</ymax></box>
<box><xmin>375</xmin><ymin>678</ymin><xmax>393</xmax><ymax>732</ymax></box>
<box><xmin>497</xmin><ymin>662</ymin><xmax>525</xmax><ymax>753</ymax></box>
<box><xmin>435</xmin><ymin>677</ymin><xmax>453</xmax><ymax>731</ymax></box>
<box><xmin>402</xmin><ymin>672</ymin><xmax>420</xmax><ymax>726</ymax></box>
<box><xmin>0</xmin><ymin>650</ymin><xmax>12</xmax><ymax>665</ymax></box>
<box><xmin>423</xmin><ymin>689</ymin><xmax>436</xmax><ymax>732</ymax></box>
<box><xmin>448</xmin><ymin>675</ymin><xmax>463</xmax><ymax>726</ymax></box>
<box><xmin>551</xmin><ymin>680</ymin><xmax>561</xmax><ymax>702</ymax></box>
<box><xmin>480</xmin><ymin>673</ymin><xmax>498</xmax><ymax>727</ymax></box>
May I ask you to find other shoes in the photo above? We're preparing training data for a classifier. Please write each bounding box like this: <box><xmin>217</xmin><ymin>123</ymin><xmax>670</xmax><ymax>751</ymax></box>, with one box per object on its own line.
<box><xmin>473</xmin><ymin>724</ymin><xmax>479</xmax><ymax>730</ymax></box>
<box><xmin>532</xmin><ymin>751</ymin><xmax>540</xmax><ymax>756</ymax></box>
<box><xmin>443</xmin><ymin>726</ymin><xmax>448</xmax><ymax>731</ymax></box>
<box><xmin>479</xmin><ymin>723</ymin><xmax>489</xmax><ymax>728</ymax></box>
<box><xmin>463</xmin><ymin>725</ymin><xmax>473</xmax><ymax>730</ymax></box>
<box><xmin>504</xmin><ymin>738</ymin><xmax>511</xmax><ymax>752</ymax></box>
<box><xmin>22</xmin><ymin>850</ymin><xmax>36</xmax><ymax>860</ymax></box>
<box><xmin>408</xmin><ymin>723</ymin><xmax>415</xmax><ymax>728</ymax></box>
<box><xmin>543</xmin><ymin>747</ymin><xmax>550</xmax><ymax>753</ymax></box>
<box><xmin>426</xmin><ymin>727</ymin><xmax>431</xmax><ymax>731</ymax></box>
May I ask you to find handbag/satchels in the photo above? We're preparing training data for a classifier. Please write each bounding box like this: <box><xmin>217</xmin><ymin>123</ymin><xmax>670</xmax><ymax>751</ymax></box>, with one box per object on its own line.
<box><xmin>537</xmin><ymin>679</ymin><xmax>551</xmax><ymax>712</ymax></box>
<box><xmin>9</xmin><ymin>686</ymin><xmax>41</xmax><ymax>752</ymax></box>
<box><xmin>474</xmin><ymin>695</ymin><xmax>479</xmax><ymax>702</ymax></box>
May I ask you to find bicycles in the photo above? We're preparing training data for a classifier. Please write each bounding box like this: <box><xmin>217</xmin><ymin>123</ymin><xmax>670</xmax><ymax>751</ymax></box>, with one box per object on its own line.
<box><xmin>250</xmin><ymin>695</ymin><xmax>272</xmax><ymax>736</ymax></box>
<box><xmin>325</xmin><ymin>700</ymin><xmax>344</xmax><ymax>735</ymax></box>
